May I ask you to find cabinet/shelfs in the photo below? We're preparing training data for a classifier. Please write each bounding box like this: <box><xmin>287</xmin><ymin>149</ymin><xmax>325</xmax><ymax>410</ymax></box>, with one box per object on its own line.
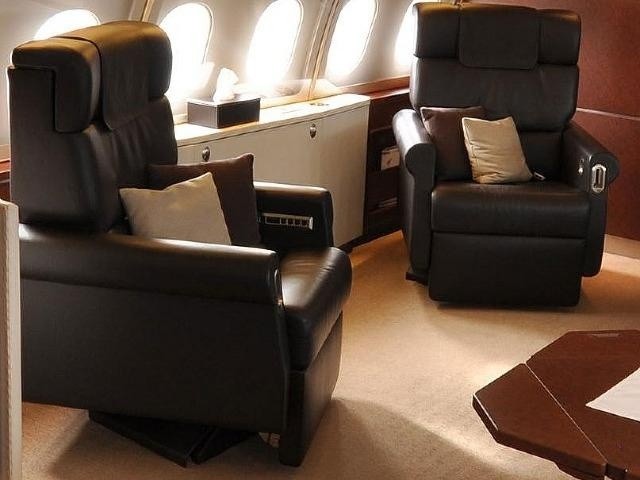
<box><xmin>175</xmin><ymin>94</ymin><xmax>372</xmax><ymax>248</ymax></box>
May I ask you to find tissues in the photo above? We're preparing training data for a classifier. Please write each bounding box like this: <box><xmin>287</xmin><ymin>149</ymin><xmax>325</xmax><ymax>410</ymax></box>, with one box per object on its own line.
<box><xmin>187</xmin><ymin>67</ymin><xmax>261</xmax><ymax>129</ymax></box>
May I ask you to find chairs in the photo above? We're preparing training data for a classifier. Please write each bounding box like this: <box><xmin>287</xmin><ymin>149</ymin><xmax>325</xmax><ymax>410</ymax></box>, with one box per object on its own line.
<box><xmin>392</xmin><ymin>2</ymin><xmax>622</xmax><ymax>310</ymax></box>
<box><xmin>6</xmin><ymin>22</ymin><xmax>352</xmax><ymax>467</ymax></box>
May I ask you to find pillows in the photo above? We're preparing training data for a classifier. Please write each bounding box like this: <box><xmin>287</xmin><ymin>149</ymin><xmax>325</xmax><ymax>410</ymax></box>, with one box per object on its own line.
<box><xmin>420</xmin><ymin>105</ymin><xmax>489</xmax><ymax>179</ymax></box>
<box><xmin>145</xmin><ymin>153</ymin><xmax>261</xmax><ymax>251</ymax></box>
<box><xmin>461</xmin><ymin>116</ymin><xmax>534</xmax><ymax>184</ymax></box>
<box><xmin>119</xmin><ymin>171</ymin><xmax>231</xmax><ymax>247</ymax></box>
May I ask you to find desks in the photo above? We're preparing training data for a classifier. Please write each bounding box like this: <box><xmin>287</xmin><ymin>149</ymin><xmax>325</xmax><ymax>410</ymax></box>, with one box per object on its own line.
<box><xmin>472</xmin><ymin>328</ymin><xmax>639</xmax><ymax>480</ymax></box>
